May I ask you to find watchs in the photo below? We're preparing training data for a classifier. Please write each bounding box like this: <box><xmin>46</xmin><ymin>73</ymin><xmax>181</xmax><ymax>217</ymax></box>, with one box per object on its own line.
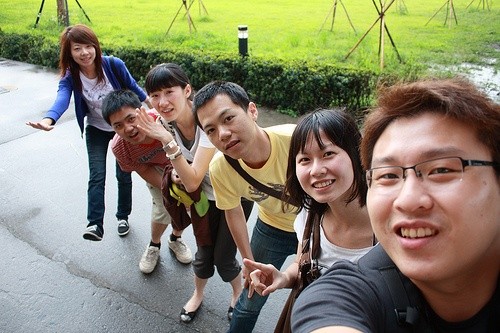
<box><xmin>162</xmin><ymin>139</ymin><xmax>177</xmax><ymax>152</ymax></box>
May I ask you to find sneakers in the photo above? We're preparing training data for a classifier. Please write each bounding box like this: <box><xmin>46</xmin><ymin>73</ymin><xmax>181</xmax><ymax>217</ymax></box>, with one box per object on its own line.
<box><xmin>83</xmin><ymin>224</ymin><xmax>103</xmax><ymax>241</ymax></box>
<box><xmin>118</xmin><ymin>218</ymin><xmax>130</xmax><ymax>235</ymax></box>
<box><xmin>168</xmin><ymin>238</ymin><xmax>192</xmax><ymax>264</ymax></box>
<box><xmin>139</xmin><ymin>240</ymin><xmax>160</xmax><ymax>273</ymax></box>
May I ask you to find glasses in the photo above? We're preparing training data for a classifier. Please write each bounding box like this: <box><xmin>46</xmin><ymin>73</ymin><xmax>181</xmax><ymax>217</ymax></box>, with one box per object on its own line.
<box><xmin>366</xmin><ymin>155</ymin><xmax>497</xmax><ymax>193</ymax></box>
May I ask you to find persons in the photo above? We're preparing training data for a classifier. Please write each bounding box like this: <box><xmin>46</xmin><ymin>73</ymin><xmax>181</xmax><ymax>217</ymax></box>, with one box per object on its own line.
<box><xmin>135</xmin><ymin>63</ymin><xmax>245</xmax><ymax>323</ymax></box>
<box><xmin>25</xmin><ymin>24</ymin><xmax>155</xmax><ymax>241</ymax></box>
<box><xmin>290</xmin><ymin>78</ymin><xmax>500</xmax><ymax>333</ymax></box>
<box><xmin>243</xmin><ymin>109</ymin><xmax>379</xmax><ymax>333</ymax></box>
<box><xmin>191</xmin><ymin>80</ymin><xmax>299</xmax><ymax>333</ymax></box>
<box><xmin>102</xmin><ymin>90</ymin><xmax>193</xmax><ymax>274</ymax></box>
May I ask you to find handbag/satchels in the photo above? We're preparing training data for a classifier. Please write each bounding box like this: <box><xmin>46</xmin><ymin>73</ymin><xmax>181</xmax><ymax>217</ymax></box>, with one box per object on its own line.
<box><xmin>166</xmin><ymin>169</ymin><xmax>194</xmax><ymax>207</ymax></box>
<box><xmin>274</xmin><ymin>203</ymin><xmax>322</xmax><ymax>333</ymax></box>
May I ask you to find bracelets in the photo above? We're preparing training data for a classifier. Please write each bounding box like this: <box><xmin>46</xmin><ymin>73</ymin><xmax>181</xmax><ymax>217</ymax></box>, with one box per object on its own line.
<box><xmin>165</xmin><ymin>145</ymin><xmax>182</xmax><ymax>160</ymax></box>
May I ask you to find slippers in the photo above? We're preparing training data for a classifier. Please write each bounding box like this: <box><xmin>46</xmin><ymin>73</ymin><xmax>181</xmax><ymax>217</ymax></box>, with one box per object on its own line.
<box><xmin>228</xmin><ymin>306</ymin><xmax>234</xmax><ymax>322</ymax></box>
<box><xmin>180</xmin><ymin>301</ymin><xmax>203</xmax><ymax>324</ymax></box>
